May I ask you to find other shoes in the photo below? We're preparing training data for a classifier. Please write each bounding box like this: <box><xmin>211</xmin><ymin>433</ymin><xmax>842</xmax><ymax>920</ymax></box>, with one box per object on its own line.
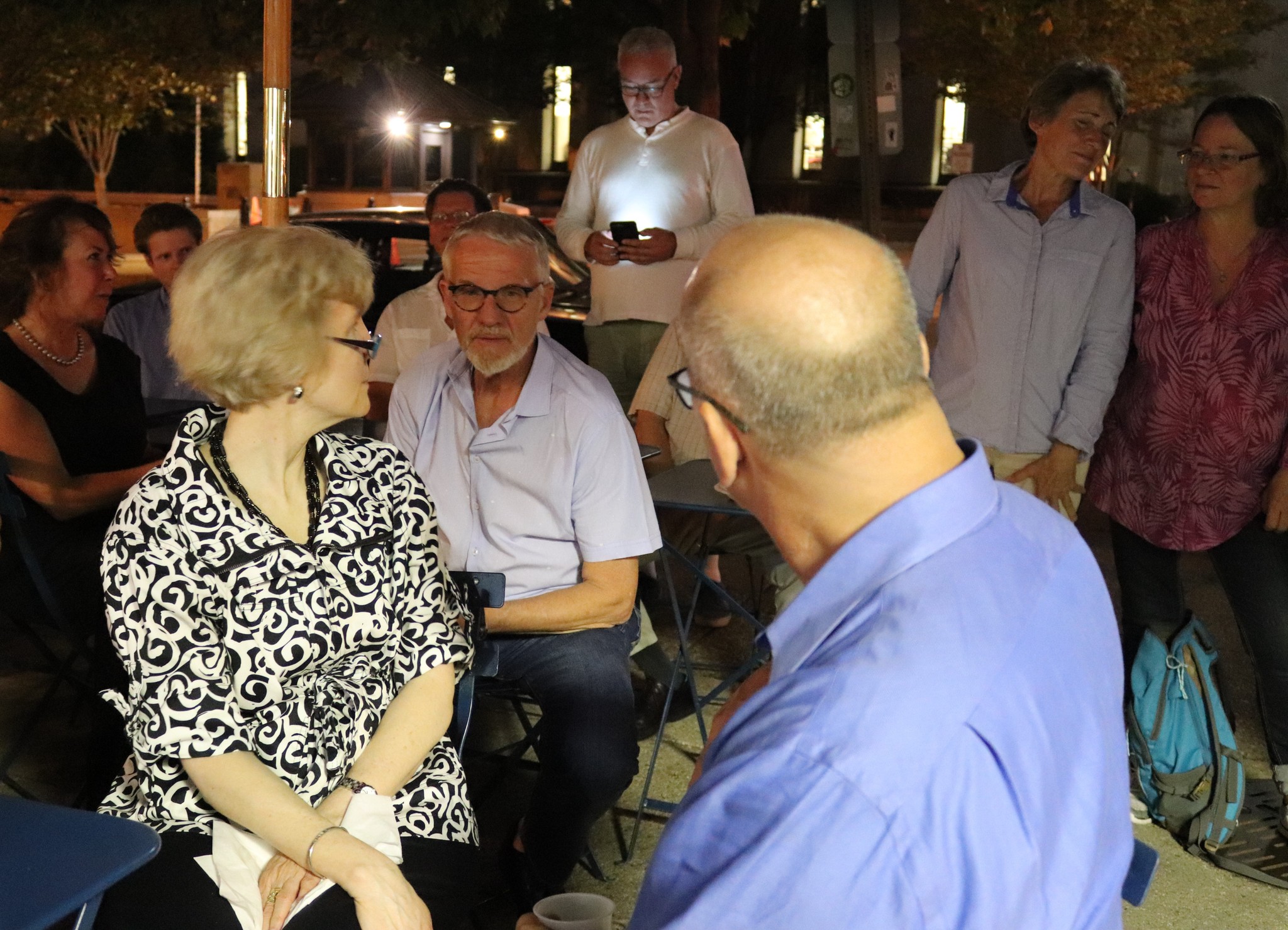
<box><xmin>499</xmin><ymin>818</ymin><xmax>566</xmax><ymax>915</ymax></box>
<box><xmin>1277</xmin><ymin>797</ymin><xmax>1288</xmax><ymax>837</ymax></box>
<box><xmin>1129</xmin><ymin>767</ymin><xmax>1153</xmax><ymax>825</ymax></box>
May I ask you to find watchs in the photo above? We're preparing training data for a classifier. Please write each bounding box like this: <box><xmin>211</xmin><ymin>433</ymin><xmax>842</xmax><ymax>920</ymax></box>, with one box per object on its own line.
<box><xmin>333</xmin><ymin>777</ymin><xmax>378</xmax><ymax>798</ymax></box>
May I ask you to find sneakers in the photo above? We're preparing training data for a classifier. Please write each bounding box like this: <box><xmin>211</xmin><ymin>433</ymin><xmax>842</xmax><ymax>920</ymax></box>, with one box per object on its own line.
<box><xmin>636</xmin><ymin>572</ymin><xmax>660</xmax><ymax>623</ymax></box>
<box><xmin>693</xmin><ymin>574</ymin><xmax>732</xmax><ymax>626</ymax></box>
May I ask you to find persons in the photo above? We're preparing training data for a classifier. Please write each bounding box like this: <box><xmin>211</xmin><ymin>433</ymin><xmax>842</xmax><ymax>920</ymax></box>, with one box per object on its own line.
<box><xmin>553</xmin><ymin>27</ymin><xmax>754</xmax><ymax>421</ymax></box>
<box><xmin>0</xmin><ymin>88</ymin><xmax>1288</xmax><ymax>930</ymax></box>
<box><xmin>904</xmin><ymin>58</ymin><xmax>1135</xmax><ymax>524</ymax></box>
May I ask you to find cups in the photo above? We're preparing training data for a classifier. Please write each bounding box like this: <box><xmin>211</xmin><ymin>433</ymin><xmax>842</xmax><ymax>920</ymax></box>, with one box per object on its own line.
<box><xmin>533</xmin><ymin>892</ymin><xmax>616</xmax><ymax>930</ymax></box>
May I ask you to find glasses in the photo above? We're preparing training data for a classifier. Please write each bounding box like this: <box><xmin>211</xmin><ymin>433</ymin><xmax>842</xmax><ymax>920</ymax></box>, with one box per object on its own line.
<box><xmin>448</xmin><ymin>281</ymin><xmax>544</xmax><ymax>313</ymax></box>
<box><xmin>666</xmin><ymin>366</ymin><xmax>750</xmax><ymax>436</ymax></box>
<box><xmin>428</xmin><ymin>210</ymin><xmax>473</xmax><ymax>230</ymax></box>
<box><xmin>619</xmin><ymin>66</ymin><xmax>677</xmax><ymax>98</ymax></box>
<box><xmin>1175</xmin><ymin>148</ymin><xmax>1261</xmax><ymax>171</ymax></box>
<box><xmin>322</xmin><ymin>329</ymin><xmax>382</xmax><ymax>360</ymax></box>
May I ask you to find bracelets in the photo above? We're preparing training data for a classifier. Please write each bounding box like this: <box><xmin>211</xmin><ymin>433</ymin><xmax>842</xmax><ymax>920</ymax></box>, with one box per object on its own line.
<box><xmin>307</xmin><ymin>826</ymin><xmax>349</xmax><ymax>879</ymax></box>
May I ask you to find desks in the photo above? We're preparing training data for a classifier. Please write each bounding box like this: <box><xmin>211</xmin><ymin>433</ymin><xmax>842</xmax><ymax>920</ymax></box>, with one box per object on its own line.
<box><xmin>629</xmin><ymin>459</ymin><xmax>765</xmax><ymax>854</ymax></box>
<box><xmin>0</xmin><ymin>796</ymin><xmax>162</xmax><ymax>930</ymax></box>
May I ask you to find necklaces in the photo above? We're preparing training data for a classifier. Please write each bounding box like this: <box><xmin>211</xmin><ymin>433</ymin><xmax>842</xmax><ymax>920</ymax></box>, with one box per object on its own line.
<box><xmin>209</xmin><ymin>419</ymin><xmax>322</xmax><ymax>549</ymax></box>
<box><xmin>13</xmin><ymin>317</ymin><xmax>83</xmax><ymax>364</ymax></box>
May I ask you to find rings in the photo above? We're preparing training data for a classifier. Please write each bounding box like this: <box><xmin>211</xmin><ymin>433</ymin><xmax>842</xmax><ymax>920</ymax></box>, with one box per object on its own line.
<box><xmin>266</xmin><ymin>888</ymin><xmax>282</xmax><ymax>904</ymax></box>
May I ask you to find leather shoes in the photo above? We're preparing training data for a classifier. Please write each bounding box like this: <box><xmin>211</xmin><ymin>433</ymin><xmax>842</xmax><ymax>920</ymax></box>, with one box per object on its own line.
<box><xmin>631</xmin><ymin>670</ymin><xmax>696</xmax><ymax>741</ymax></box>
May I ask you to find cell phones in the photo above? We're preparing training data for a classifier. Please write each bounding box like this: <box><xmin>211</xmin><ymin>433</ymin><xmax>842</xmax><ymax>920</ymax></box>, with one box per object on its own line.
<box><xmin>610</xmin><ymin>222</ymin><xmax>639</xmax><ymax>254</ymax></box>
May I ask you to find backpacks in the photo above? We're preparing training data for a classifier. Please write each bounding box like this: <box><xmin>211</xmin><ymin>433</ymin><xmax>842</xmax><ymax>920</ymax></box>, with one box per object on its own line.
<box><xmin>1129</xmin><ymin>605</ymin><xmax>1245</xmax><ymax>858</ymax></box>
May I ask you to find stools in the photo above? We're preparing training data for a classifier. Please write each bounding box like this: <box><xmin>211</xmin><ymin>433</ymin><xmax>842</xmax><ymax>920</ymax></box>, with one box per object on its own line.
<box><xmin>475</xmin><ymin>606</ymin><xmax>642</xmax><ymax>881</ymax></box>
